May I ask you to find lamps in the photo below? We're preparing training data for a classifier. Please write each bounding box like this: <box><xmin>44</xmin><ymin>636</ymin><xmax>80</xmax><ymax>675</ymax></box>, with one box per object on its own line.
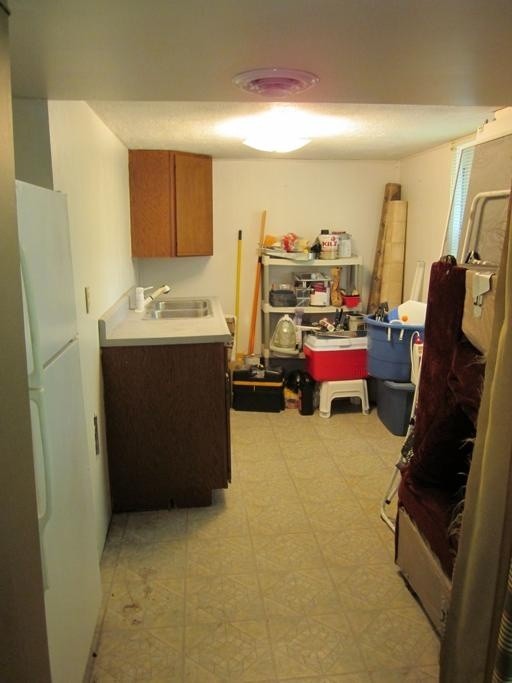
<box><xmin>231</xmin><ymin>66</ymin><xmax>321</xmax><ymax>100</ymax></box>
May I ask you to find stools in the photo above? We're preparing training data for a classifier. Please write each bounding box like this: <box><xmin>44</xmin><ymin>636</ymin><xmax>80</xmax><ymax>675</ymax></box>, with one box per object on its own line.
<box><xmin>318</xmin><ymin>379</ymin><xmax>370</xmax><ymax>419</ymax></box>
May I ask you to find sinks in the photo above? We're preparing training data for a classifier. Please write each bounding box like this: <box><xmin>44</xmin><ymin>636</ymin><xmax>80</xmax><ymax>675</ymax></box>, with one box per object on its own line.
<box><xmin>151</xmin><ymin>310</ymin><xmax>214</xmax><ymax>319</ymax></box>
<box><xmin>153</xmin><ymin>298</ymin><xmax>214</xmax><ymax>310</ymax></box>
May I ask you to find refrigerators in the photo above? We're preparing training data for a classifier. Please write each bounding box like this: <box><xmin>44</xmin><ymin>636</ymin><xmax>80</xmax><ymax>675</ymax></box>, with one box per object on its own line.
<box><xmin>0</xmin><ymin>178</ymin><xmax>107</xmax><ymax>683</ymax></box>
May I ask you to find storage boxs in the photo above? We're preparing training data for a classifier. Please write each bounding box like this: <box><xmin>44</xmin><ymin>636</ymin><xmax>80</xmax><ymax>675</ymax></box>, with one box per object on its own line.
<box><xmin>303</xmin><ymin>334</ymin><xmax>369</xmax><ymax>383</ymax></box>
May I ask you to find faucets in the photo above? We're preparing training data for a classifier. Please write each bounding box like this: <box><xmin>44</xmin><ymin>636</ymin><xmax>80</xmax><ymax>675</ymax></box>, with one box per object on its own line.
<box><xmin>136</xmin><ymin>285</ymin><xmax>170</xmax><ymax>312</ymax></box>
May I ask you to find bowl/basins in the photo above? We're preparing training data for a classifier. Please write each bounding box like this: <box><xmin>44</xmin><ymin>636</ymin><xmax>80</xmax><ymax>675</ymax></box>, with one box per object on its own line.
<box><xmin>342</xmin><ymin>296</ymin><xmax>360</xmax><ymax>306</ymax></box>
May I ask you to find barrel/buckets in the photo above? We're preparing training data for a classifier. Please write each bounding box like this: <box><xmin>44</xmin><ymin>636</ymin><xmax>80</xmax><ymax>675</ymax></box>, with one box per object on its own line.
<box><xmin>319</xmin><ymin>235</ymin><xmax>339</xmax><ymax>260</ymax></box>
<box><xmin>396</xmin><ymin>300</ymin><xmax>427</xmax><ymax>327</ymax></box>
<box><xmin>377</xmin><ymin>380</ymin><xmax>415</xmax><ymax>436</ymax></box>
<box><xmin>364</xmin><ymin>314</ymin><xmax>425</xmax><ymax>382</ymax></box>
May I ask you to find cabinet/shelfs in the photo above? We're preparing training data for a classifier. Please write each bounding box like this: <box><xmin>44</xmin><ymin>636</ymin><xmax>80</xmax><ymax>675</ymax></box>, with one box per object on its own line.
<box><xmin>128</xmin><ymin>149</ymin><xmax>214</xmax><ymax>258</ymax></box>
<box><xmin>259</xmin><ymin>252</ymin><xmax>363</xmax><ymax>370</ymax></box>
